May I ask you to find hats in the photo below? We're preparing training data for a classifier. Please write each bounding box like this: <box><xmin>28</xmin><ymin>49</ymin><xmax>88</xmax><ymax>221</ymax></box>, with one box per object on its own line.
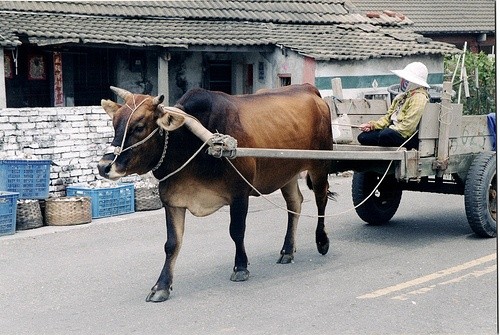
<box><xmin>390</xmin><ymin>62</ymin><xmax>430</xmax><ymax>88</ymax></box>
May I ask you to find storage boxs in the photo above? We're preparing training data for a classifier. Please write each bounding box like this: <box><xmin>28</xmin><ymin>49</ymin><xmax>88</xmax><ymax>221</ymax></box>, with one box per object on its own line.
<box><xmin>0</xmin><ymin>158</ymin><xmax>52</xmax><ymax>199</ymax></box>
<box><xmin>66</xmin><ymin>181</ymin><xmax>136</xmax><ymax>220</ymax></box>
<box><xmin>0</xmin><ymin>190</ymin><xmax>20</xmax><ymax>237</ymax></box>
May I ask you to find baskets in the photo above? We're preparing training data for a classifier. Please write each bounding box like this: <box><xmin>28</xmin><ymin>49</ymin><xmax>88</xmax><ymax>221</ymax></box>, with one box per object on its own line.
<box><xmin>45</xmin><ymin>196</ymin><xmax>92</xmax><ymax>226</ymax></box>
<box><xmin>16</xmin><ymin>199</ymin><xmax>43</xmax><ymax>230</ymax></box>
<box><xmin>134</xmin><ymin>184</ymin><xmax>163</xmax><ymax>211</ymax></box>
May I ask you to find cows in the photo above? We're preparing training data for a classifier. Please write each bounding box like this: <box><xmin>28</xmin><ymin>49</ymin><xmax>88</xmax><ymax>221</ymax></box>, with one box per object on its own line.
<box><xmin>98</xmin><ymin>82</ymin><xmax>333</xmax><ymax>303</ymax></box>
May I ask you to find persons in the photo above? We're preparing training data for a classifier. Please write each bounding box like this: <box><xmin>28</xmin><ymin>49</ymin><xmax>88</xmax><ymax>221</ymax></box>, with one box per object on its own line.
<box><xmin>358</xmin><ymin>62</ymin><xmax>430</xmax><ymax>151</ymax></box>
